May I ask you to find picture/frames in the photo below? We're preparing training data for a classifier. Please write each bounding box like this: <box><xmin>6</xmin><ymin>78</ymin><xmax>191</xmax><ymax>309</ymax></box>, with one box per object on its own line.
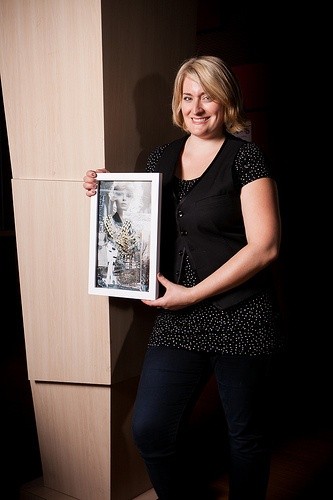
<box><xmin>88</xmin><ymin>173</ymin><xmax>162</xmax><ymax>300</ymax></box>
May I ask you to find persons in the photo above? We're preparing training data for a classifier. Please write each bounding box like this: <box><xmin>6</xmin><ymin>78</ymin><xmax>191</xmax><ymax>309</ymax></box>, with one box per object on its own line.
<box><xmin>84</xmin><ymin>57</ymin><xmax>281</xmax><ymax>500</ymax></box>
<box><xmin>98</xmin><ymin>180</ymin><xmax>150</xmax><ymax>291</ymax></box>
